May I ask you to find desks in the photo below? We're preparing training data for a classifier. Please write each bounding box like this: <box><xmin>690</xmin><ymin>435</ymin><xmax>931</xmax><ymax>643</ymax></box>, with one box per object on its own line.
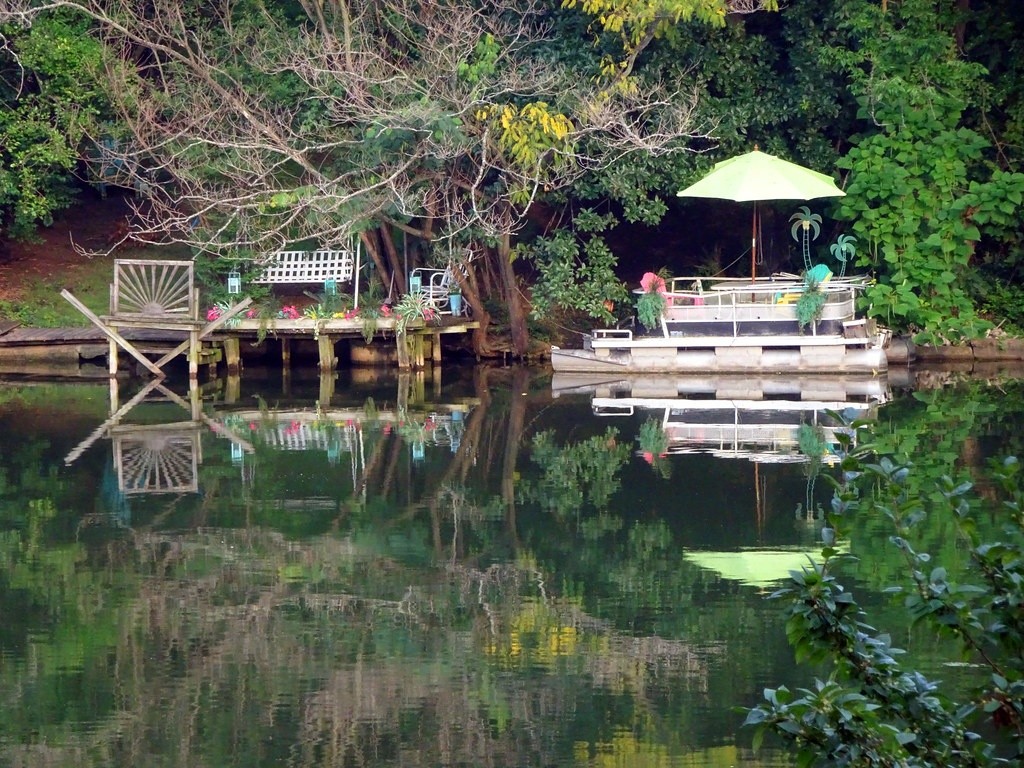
<box><xmin>711</xmin><ymin>281</ymin><xmax>796</xmax><ymax>306</ymax></box>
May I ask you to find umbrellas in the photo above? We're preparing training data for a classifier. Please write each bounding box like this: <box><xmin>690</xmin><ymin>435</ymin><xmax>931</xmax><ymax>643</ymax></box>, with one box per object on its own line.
<box><xmin>683</xmin><ymin>462</ymin><xmax>850</xmax><ymax>592</ymax></box>
<box><xmin>677</xmin><ymin>144</ymin><xmax>846</xmax><ymax>303</ymax></box>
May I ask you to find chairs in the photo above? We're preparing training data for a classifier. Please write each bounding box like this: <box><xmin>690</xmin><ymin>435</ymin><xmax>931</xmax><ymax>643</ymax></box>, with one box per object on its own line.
<box><xmin>775</xmin><ymin>264</ymin><xmax>832</xmax><ymax>305</ymax></box>
<box><xmin>639</xmin><ymin>273</ymin><xmax>703</xmax><ymax>305</ymax></box>
<box><xmin>409</xmin><ymin>248</ymin><xmax>473</xmax><ymax>314</ymax></box>
